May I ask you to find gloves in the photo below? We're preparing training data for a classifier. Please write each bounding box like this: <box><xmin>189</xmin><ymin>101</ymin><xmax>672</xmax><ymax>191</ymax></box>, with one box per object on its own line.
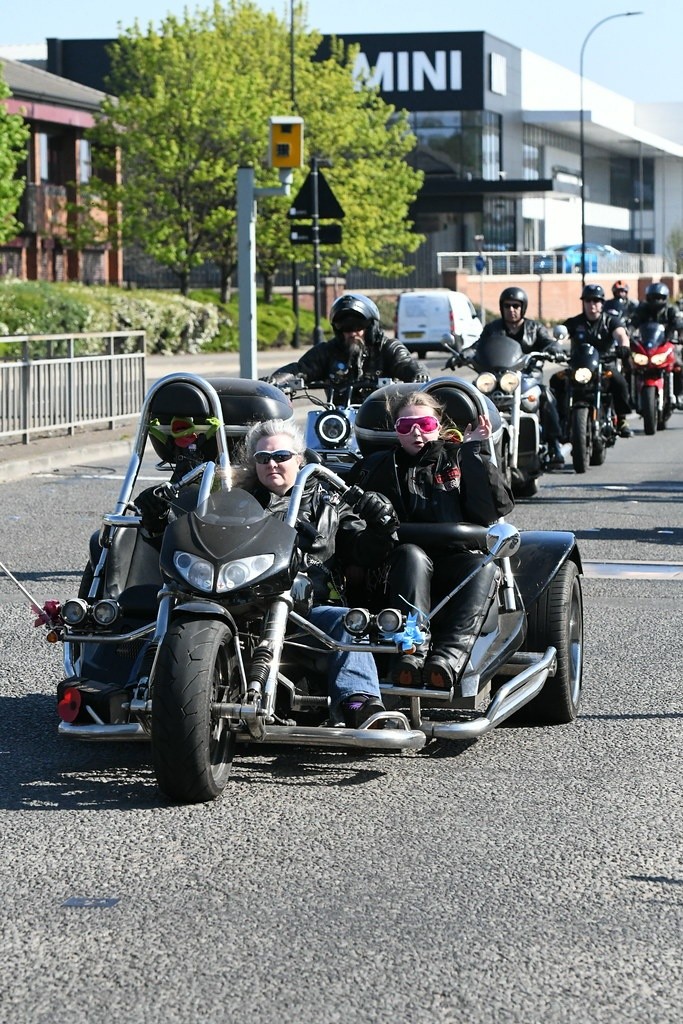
<box><xmin>258</xmin><ymin>376</ymin><xmax>275</xmax><ymax>384</ymax></box>
<box><xmin>445</xmin><ymin>355</ymin><xmax>462</xmax><ymax>372</ymax></box>
<box><xmin>133</xmin><ymin>486</ymin><xmax>169</xmax><ymax>535</ymax></box>
<box><xmin>411</xmin><ymin>368</ymin><xmax>431</xmax><ymax>384</ymax></box>
<box><xmin>552</xmin><ymin>348</ymin><xmax>567</xmax><ymax>364</ymax></box>
<box><xmin>352</xmin><ymin>491</ymin><xmax>396</xmax><ymax>527</ymax></box>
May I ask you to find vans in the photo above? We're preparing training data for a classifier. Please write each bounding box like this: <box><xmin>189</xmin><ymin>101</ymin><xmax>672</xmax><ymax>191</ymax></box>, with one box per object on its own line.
<box><xmin>394</xmin><ymin>291</ymin><xmax>483</xmax><ymax>360</ymax></box>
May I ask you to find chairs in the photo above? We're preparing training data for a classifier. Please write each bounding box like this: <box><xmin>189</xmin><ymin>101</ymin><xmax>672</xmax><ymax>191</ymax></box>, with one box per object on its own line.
<box><xmin>384</xmin><ymin>377</ymin><xmax>502</xmax><ymax>590</ymax></box>
<box><xmin>90</xmin><ymin>372</ymin><xmax>233</xmax><ymax>622</ymax></box>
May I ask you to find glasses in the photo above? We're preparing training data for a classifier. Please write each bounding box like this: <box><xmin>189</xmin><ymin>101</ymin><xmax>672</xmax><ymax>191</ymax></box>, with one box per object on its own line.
<box><xmin>501</xmin><ymin>303</ymin><xmax>521</xmax><ymax>309</ymax></box>
<box><xmin>393</xmin><ymin>416</ymin><xmax>442</xmax><ymax>436</ymax></box>
<box><xmin>251</xmin><ymin>449</ymin><xmax>298</xmax><ymax>465</ymax></box>
<box><xmin>331</xmin><ymin>317</ymin><xmax>371</xmax><ymax>331</ymax></box>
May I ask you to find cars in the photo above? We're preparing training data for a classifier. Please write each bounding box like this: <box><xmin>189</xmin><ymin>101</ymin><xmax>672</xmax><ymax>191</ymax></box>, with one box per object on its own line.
<box><xmin>533</xmin><ymin>244</ymin><xmax>622</xmax><ymax>272</ymax></box>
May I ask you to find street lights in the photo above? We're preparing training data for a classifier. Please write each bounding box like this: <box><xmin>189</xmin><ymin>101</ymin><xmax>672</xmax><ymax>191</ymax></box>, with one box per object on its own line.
<box><xmin>579</xmin><ymin>11</ymin><xmax>641</xmax><ymax>290</ymax></box>
<box><xmin>308</xmin><ymin>157</ymin><xmax>334</xmax><ymax>345</ymax></box>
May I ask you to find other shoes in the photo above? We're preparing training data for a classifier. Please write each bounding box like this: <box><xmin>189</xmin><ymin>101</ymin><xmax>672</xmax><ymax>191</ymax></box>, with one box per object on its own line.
<box><xmin>621</xmin><ymin>425</ymin><xmax>632</xmax><ymax>438</ymax></box>
<box><xmin>549</xmin><ymin>455</ymin><xmax>566</xmax><ymax>470</ymax></box>
<box><xmin>394</xmin><ymin>653</ymin><xmax>454</xmax><ymax>702</ymax></box>
<box><xmin>676</xmin><ymin>394</ymin><xmax>683</xmax><ymax>409</ymax></box>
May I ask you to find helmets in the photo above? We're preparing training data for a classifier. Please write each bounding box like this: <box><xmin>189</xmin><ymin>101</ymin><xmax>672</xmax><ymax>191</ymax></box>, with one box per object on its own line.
<box><xmin>644</xmin><ymin>282</ymin><xmax>670</xmax><ymax>299</ymax></box>
<box><xmin>330</xmin><ymin>293</ymin><xmax>381</xmax><ymax>327</ymax></box>
<box><xmin>499</xmin><ymin>287</ymin><xmax>528</xmax><ymax>314</ymax></box>
<box><xmin>580</xmin><ymin>285</ymin><xmax>605</xmax><ymax>299</ymax></box>
<box><xmin>612</xmin><ymin>280</ymin><xmax>629</xmax><ymax>293</ymax></box>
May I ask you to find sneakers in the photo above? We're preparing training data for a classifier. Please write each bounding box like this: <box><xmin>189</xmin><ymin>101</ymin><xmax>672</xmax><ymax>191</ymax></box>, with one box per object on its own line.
<box><xmin>344</xmin><ymin>693</ymin><xmax>387</xmax><ymax>730</ymax></box>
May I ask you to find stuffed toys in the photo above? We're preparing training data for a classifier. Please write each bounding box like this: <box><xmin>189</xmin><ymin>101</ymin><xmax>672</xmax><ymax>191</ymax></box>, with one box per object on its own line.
<box><xmin>149</xmin><ymin>414</ymin><xmax>221</xmax><ymax>484</ymax></box>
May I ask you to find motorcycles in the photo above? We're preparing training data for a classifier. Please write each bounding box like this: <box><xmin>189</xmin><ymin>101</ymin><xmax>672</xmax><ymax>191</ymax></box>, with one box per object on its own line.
<box><xmin>274</xmin><ymin>365</ymin><xmax>504</xmax><ymax>490</ymax></box>
<box><xmin>630</xmin><ymin>322</ymin><xmax>677</xmax><ymax>435</ymax></box>
<box><xmin>441</xmin><ymin>327</ymin><xmax>568</xmax><ymax>499</ymax></box>
<box><xmin>47</xmin><ymin>373</ymin><xmax>583</xmax><ymax>801</ymax></box>
<box><xmin>555</xmin><ymin>342</ymin><xmax>620</xmax><ymax>474</ymax></box>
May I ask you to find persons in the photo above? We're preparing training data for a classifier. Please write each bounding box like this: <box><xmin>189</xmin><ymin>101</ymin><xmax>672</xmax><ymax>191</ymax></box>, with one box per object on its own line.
<box><xmin>550</xmin><ymin>284</ymin><xmax>634</xmax><ymax>438</ymax></box>
<box><xmin>445</xmin><ymin>286</ymin><xmax>567</xmax><ymax>464</ymax></box>
<box><xmin>604</xmin><ymin>280</ymin><xmax>636</xmax><ymax>325</ymax></box>
<box><xmin>259</xmin><ymin>293</ymin><xmax>430</xmax><ymax>409</ymax></box>
<box><xmin>627</xmin><ymin>283</ymin><xmax>683</xmax><ymax>411</ymax></box>
<box><xmin>345</xmin><ymin>388</ymin><xmax>516</xmax><ymax>691</ymax></box>
<box><xmin>124</xmin><ymin>416</ymin><xmax>401</xmax><ymax>729</ymax></box>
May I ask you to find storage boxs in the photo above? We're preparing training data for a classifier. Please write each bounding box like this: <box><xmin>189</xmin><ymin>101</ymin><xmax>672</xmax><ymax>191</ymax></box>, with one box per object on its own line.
<box><xmin>352</xmin><ymin>382</ymin><xmax>502</xmax><ymax>463</ymax></box>
<box><xmin>149</xmin><ymin>377</ymin><xmax>296</xmax><ymax>463</ymax></box>
